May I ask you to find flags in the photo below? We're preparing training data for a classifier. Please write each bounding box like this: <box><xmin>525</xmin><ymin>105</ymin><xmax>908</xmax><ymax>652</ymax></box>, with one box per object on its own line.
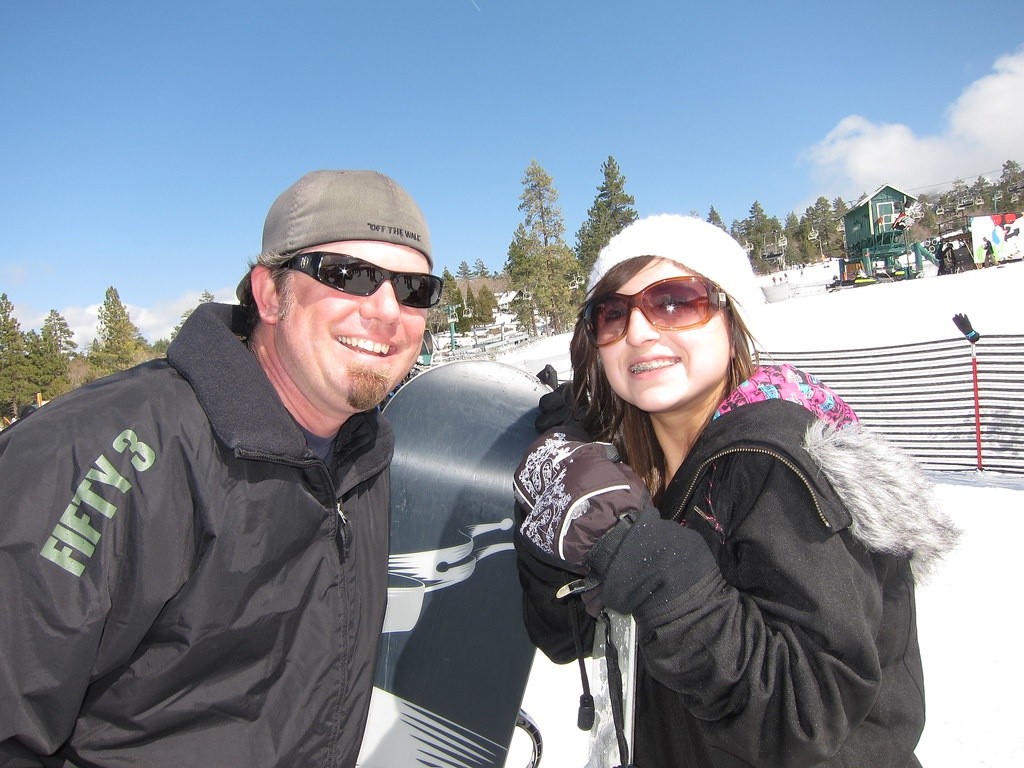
<box><xmin>894</xmin><ymin>214</ymin><xmax>915</xmax><ymax>230</ymax></box>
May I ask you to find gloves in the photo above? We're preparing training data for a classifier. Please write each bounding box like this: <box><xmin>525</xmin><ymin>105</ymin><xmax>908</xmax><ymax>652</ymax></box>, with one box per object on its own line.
<box><xmin>512</xmin><ymin>423</ymin><xmax>655</xmax><ymax>618</ymax></box>
<box><xmin>952</xmin><ymin>313</ymin><xmax>980</xmax><ymax>343</ymax></box>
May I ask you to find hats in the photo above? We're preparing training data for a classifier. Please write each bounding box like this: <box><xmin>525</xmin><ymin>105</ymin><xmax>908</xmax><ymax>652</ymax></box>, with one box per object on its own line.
<box><xmin>235</xmin><ymin>169</ymin><xmax>434</xmax><ymax>308</ymax></box>
<box><xmin>584</xmin><ymin>213</ymin><xmax>767</xmax><ymax>315</ymax></box>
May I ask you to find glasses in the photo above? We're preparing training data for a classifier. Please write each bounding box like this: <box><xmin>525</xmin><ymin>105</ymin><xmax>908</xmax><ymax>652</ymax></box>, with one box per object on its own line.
<box><xmin>581</xmin><ymin>275</ymin><xmax>730</xmax><ymax>349</ymax></box>
<box><xmin>276</xmin><ymin>250</ymin><xmax>446</xmax><ymax>308</ymax></box>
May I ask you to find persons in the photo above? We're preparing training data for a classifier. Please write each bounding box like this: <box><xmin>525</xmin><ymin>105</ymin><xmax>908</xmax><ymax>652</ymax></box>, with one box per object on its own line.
<box><xmin>0</xmin><ymin>170</ymin><xmax>432</xmax><ymax>768</ymax></box>
<box><xmin>935</xmin><ymin>243</ymin><xmax>956</xmax><ymax>276</ymax></box>
<box><xmin>772</xmin><ymin>273</ymin><xmax>788</xmax><ymax>285</ymax></box>
<box><xmin>513</xmin><ymin>213</ymin><xmax>962</xmax><ymax>768</ymax></box>
<box><xmin>983</xmin><ymin>236</ymin><xmax>1000</xmax><ymax>268</ymax></box>
